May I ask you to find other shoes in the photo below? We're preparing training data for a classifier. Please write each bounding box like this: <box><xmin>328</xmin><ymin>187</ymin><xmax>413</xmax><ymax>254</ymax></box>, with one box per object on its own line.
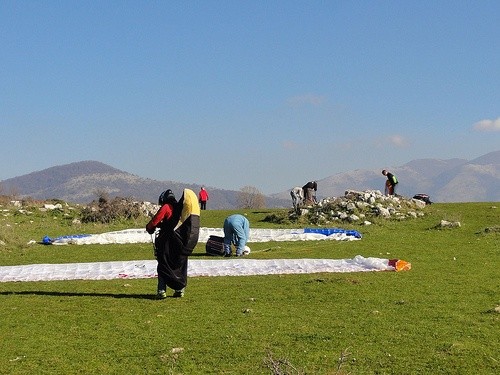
<box><xmin>155</xmin><ymin>289</ymin><xmax>167</xmax><ymax>299</ymax></box>
<box><xmin>173</xmin><ymin>292</ymin><xmax>184</xmax><ymax>297</ymax></box>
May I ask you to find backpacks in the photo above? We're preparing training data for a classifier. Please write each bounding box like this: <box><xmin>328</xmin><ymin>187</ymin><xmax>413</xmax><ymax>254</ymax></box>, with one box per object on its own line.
<box><xmin>165</xmin><ymin>188</ymin><xmax>200</xmax><ymax>256</ymax></box>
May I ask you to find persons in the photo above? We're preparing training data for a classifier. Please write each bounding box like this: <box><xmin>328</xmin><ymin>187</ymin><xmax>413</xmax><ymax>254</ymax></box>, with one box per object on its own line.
<box><xmin>146</xmin><ymin>189</ymin><xmax>188</xmax><ymax>298</ymax></box>
<box><xmin>381</xmin><ymin>169</ymin><xmax>399</xmax><ymax>196</ymax></box>
<box><xmin>198</xmin><ymin>187</ymin><xmax>209</xmax><ymax>211</ymax></box>
<box><xmin>290</xmin><ymin>181</ymin><xmax>318</xmax><ymax>210</ymax></box>
<box><xmin>223</xmin><ymin>214</ymin><xmax>249</xmax><ymax>256</ymax></box>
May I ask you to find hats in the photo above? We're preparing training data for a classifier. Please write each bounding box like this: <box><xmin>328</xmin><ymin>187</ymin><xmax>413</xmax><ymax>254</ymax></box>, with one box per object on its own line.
<box><xmin>159</xmin><ymin>189</ymin><xmax>173</xmax><ymax>204</ymax></box>
